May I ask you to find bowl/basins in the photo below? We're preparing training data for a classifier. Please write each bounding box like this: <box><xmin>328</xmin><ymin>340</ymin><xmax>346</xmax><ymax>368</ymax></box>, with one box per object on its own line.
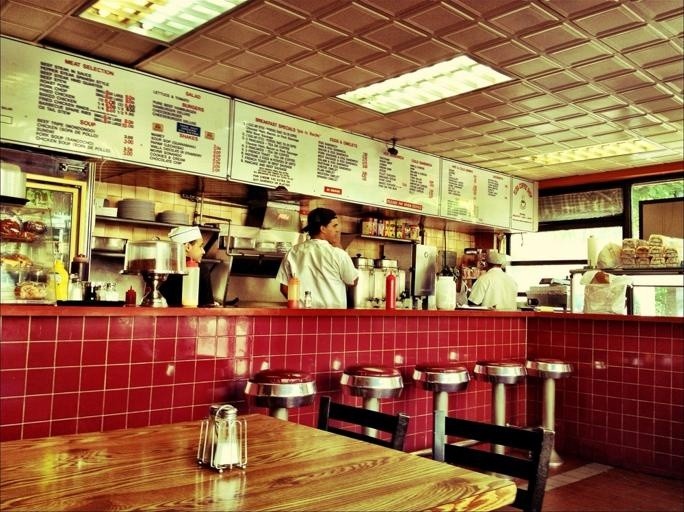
<box><xmin>464</xmin><ymin>269</ymin><xmax>486</xmax><ymax>278</ymax></box>
<box><xmin>92</xmin><ymin>237</ymin><xmax>128</xmax><ymax>254</ymax></box>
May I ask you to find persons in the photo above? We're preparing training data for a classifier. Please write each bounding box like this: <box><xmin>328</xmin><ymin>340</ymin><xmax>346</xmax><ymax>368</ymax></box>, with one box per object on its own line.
<box><xmin>466</xmin><ymin>249</ymin><xmax>517</xmax><ymax>310</ymax></box>
<box><xmin>158</xmin><ymin>225</ymin><xmax>213</xmax><ymax>308</ymax></box>
<box><xmin>274</xmin><ymin>206</ymin><xmax>358</xmax><ymax>311</ymax></box>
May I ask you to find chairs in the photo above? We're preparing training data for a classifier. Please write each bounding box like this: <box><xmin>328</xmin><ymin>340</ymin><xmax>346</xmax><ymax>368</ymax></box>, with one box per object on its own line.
<box><xmin>444</xmin><ymin>415</ymin><xmax>556</xmax><ymax>511</ymax></box>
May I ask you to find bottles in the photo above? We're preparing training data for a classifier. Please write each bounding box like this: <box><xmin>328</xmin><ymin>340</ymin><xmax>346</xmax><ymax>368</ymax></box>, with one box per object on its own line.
<box><xmin>360</xmin><ymin>217</ymin><xmax>420</xmax><ymax>241</ymax></box>
<box><xmin>202</xmin><ymin>478</ymin><xmax>238</xmax><ymax>511</ymax></box>
<box><xmin>587</xmin><ymin>235</ymin><xmax>598</xmax><ymax>266</ymax></box>
<box><xmin>303</xmin><ymin>290</ymin><xmax>311</xmax><ymax>307</ymax></box>
<box><xmin>126</xmin><ymin>285</ymin><xmax>137</xmax><ymax>306</ymax></box>
<box><xmin>384</xmin><ymin>270</ymin><xmax>396</xmax><ymax>309</ymax></box>
<box><xmin>287</xmin><ymin>271</ymin><xmax>300</xmax><ymax>308</ymax></box>
<box><xmin>436</xmin><ymin>266</ymin><xmax>458</xmax><ymax>311</ymax></box>
<box><xmin>181</xmin><ymin>256</ymin><xmax>200</xmax><ymax>307</ymax></box>
<box><xmin>207</xmin><ymin>405</ymin><xmax>242</xmax><ymax>467</ymax></box>
<box><xmin>47</xmin><ymin>254</ymin><xmax>118</xmax><ymax>301</ymax></box>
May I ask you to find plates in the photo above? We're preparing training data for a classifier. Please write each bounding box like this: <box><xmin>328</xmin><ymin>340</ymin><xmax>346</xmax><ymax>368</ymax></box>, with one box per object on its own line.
<box><xmin>115</xmin><ymin>199</ymin><xmax>190</xmax><ymax>224</ymax></box>
<box><xmin>1</xmin><ymin>194</ymin><xmax>30</xmax><ymax>206</ymax></box>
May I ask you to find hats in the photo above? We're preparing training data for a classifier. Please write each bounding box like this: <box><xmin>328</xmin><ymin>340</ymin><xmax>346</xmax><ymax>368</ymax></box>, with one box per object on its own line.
<box><xmin>167</xmin><ymin>226</ymin><xmax>202</xmax><ymax>243</ymax></box>
<box><xmin>487</xmin><ymin>252</ymin><xmax>503</xmax><ymax>264</ymax></box>
<box><xmin>298</xmin><ymin>208</ymin><xmax>336</xmax><ymax>233</ymax></box>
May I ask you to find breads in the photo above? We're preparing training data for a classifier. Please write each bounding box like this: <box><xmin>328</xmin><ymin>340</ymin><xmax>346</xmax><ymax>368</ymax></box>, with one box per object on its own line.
<box><xmin>1</xmin><ymin>254</ymin><xmax>34</xmax><ymax>270</ymax></box>
<box><xmin>620</xmin><ymin>235</ymin><xmax>684</xmax><ymax>268</ymax></box>
<box><xmin>14</xmin><ymin>286</ymin><xmax>41</xmax><ymax>299</ymax></box>
<box><xmin>1</xmin><ymin>218</ymin><xmax>47</xmax><ymax>243</ymax></box>
